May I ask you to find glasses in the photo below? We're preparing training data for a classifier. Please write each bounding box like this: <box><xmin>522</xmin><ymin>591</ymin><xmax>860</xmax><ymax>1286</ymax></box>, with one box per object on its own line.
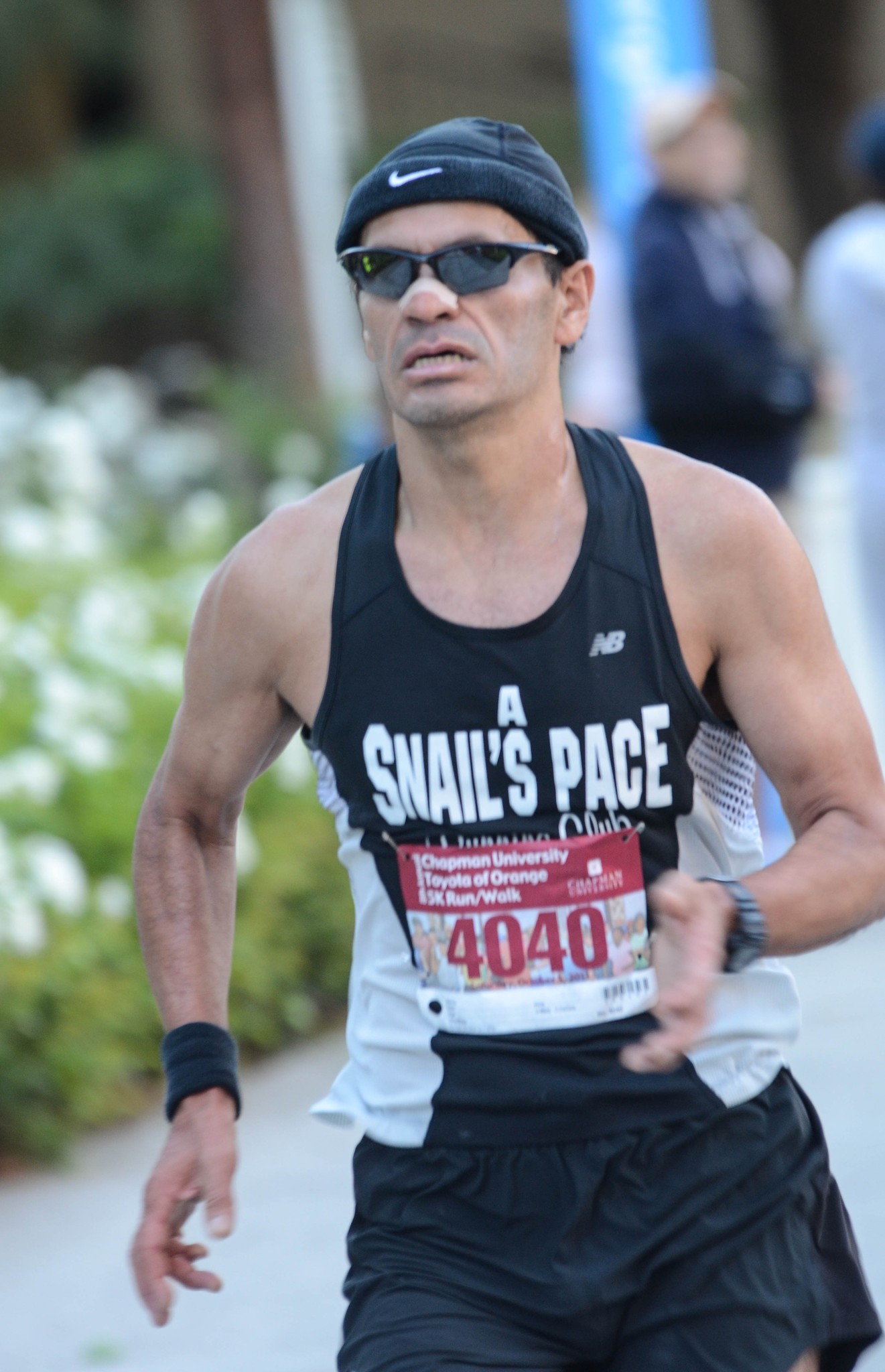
<box><xmin>339</xmin><ymin>239</ymin><xmax>562</xmax><ymax>298</ymax></box>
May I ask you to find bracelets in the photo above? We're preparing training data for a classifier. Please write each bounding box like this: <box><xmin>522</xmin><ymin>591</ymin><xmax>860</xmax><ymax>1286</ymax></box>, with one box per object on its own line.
<box><xmin>162</xmin><ymin>1022</ymin><xmax>241</xmax><ymax>1123</ymax></box>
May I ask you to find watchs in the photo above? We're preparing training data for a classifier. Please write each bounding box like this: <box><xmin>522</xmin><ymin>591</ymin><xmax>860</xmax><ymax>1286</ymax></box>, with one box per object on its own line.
<box><xmin>698</xmin><ymin>876</ymin><xmax>766</xmax><ymax>974</ymax></box>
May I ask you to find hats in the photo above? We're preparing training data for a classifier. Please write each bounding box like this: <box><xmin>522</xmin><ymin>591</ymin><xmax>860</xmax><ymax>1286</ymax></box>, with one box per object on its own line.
<box><xmin>333</xmin><ymin>115</ymin><xmax>588</xmax><ymax>257</ymax></box>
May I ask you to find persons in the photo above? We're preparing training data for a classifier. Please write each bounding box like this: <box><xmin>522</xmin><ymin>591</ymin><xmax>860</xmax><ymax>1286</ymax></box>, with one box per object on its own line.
<box><xmin>634</xmin><ymin>68</ymin><xmax>852</xmax><ymax>507</ymax></box>
<box><xmin>803</xmin><ymin>103</ymin><xmax>885</xmax><ymax>673</ymax></box>
<box><xmin>132</xmin><ymin>116</ymin><xmax>885</xmax><ymax>1372</ymax></box>
